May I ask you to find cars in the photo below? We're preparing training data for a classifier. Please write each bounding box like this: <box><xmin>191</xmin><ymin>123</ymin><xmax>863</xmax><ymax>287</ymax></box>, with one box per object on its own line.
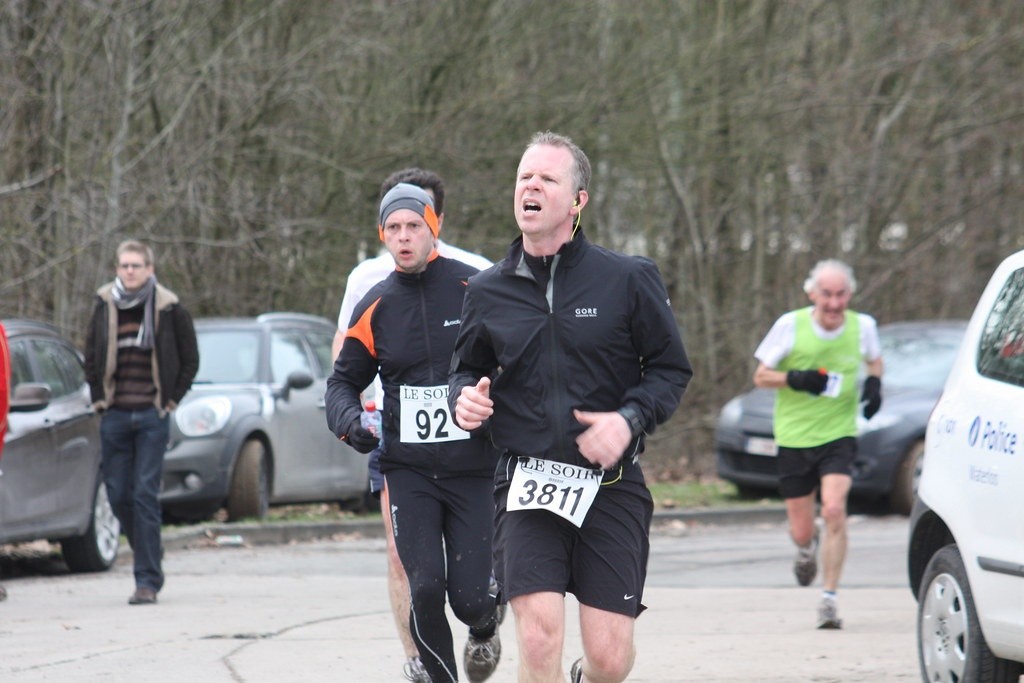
<box><xmin>148</xmin><ymin>310</ymin><xmax>387</xmax><ymax>523</ymax></box>
<box><xmin>906</xmin><ymin>247</ymin><xmax>1024</xmax><ymax>682</ymax></box>
<box><xmin>709</xmin><ymin>321</ymin><xmax>1022</xmax><ymax>518</ymax></box>
<box><xmin>0</xmin><ymin>318</ymin><xmax>124</xmax><ymax>584</ymax></box>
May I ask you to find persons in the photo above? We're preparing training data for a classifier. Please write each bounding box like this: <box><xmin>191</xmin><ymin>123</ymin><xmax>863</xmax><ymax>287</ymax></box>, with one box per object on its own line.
<box><xmin>0</xmin><ymin>323</ymin><xmax>12</xmax><ymax>603</ymax></box>
<box><xmin>325</xmin><ymin>167</ymin><xmax>507</xmax><ymax>683</ymax></box>
<box><xmin>448</xmin><ymin>128</ymin><xmax>695</xmax><ymax>683</ymax></box>
<box><xmin>85</xmin><ymin>239</ymin><xmax>200</xmax><ymax>606</ymax></box>
<box><xmin>753</xmin><ymin>259</ymin><xmax>885</xmax><ymax>629</ymax></box>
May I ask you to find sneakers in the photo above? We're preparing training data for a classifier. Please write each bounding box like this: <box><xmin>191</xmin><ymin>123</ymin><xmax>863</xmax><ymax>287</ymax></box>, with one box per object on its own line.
<box><xmin>463</xmin><ymin>594</ymin><xmax>507</xmax><ymax>683</ymax></box>
<box><xmin>402</xmin><ymin>661</ymin><xmax>432</xmax><ymax>683</ymax></box>
<box><xmin>570</xmin><ymin>656</ymin><xmax>584</xmax><ymax>683</ymax></box>
<box><xmin>489</xmin><ymin>570</ymin><xmax>497</xmax><ymax>589</ymax></box>
<box><xmin>815</xmin><ymin>602</ymin><xmax>842</xmax><ymax>629</ymax></box>
<box><xmin>789</xmin><ymin>526</ymin><xmax>821</xmax><ymax>586</ymax></box>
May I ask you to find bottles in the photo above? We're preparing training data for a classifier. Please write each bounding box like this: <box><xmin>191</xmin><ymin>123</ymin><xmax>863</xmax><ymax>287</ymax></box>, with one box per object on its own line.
<box><xmin>360</xmin><ymin>400</ymin><xmax>384</xmax><ymax>449</ymax></box>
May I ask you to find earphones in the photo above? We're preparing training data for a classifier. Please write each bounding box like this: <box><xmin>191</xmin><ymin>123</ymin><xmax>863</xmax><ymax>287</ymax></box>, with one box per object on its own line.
<box><xmin>573</xmin><ymin>193</ymin><xmax>581</xmax><ymax>206</ymax></box>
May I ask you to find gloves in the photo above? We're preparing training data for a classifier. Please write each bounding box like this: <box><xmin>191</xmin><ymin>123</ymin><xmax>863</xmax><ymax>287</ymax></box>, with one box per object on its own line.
<box><xmin>859</xmin><ymin>375</ymin><xmax>882</xmax><ymax>420</ymax></box>
<box><xmin>787</xmin><ymin>369</ymin><xmax>828</xmax><ymax>396</ymax></box>
<box><xmin>346</xmin><ymin>418</ymin><xmax>380</xmax><ymax>454</ymax></box>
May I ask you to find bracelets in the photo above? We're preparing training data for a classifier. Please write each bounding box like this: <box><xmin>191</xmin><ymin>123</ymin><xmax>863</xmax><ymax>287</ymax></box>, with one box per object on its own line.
<box><xmin>617</xmin><ymin>405</ymin><xmax>642</xmax><ymax>438</ymax></box>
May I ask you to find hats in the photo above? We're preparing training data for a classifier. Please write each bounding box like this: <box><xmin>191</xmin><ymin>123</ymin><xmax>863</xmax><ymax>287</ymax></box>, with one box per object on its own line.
<box><xmin>379</xmin><ymin>181</ymin><xmax>439</xmax><ymax>242</ymax></box>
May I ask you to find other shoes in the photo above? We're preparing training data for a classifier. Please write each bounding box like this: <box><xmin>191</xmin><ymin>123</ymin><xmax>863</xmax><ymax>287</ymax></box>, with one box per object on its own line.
<box><xmin>0</xmin><ymin>584</ymin><xmax>7</xmax><ymax>602</ymax></box>
<box><xmin>135</xmin><ymin>587</ymin><xmax>157</xmax><ymax>604</ymax></box>
<box><xmin>128</xmin><ymin>592</ymin><xmax>139</xmax><ymax>605</ymax></box>
